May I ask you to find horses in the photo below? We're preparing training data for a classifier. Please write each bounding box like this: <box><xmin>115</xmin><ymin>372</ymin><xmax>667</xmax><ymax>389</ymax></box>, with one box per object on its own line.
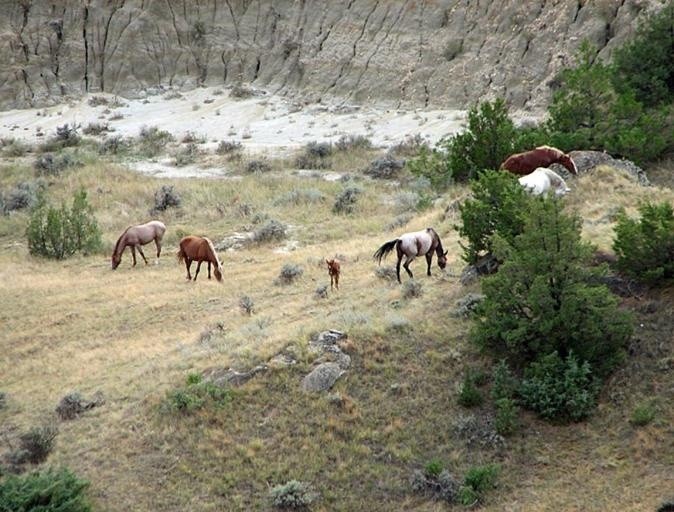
<box><xmin>518</xmin><ymin>167</ymin><xmax>572</xmax><ymax>201</ymax></box>
<box><xmin>326</xmin><ymin>259</ymin><xmax>341</xmax><ymax>291</ymax></box>
<box><xmin>500</xmin><ymin>145</ymin><xmax>578</xmax><ymax>174</ymax></box>
<box><xmin>112</xmin><ymin>221</ymin><xmax>166</xmax><ymax>270</ymax></box>
<box><xmin>176</xmin><ymin>236</ymin><xmax>224</xmax><ymax>282</ymax></box>
<box><xmin>372</xmin><ymin>228</ymin><xmax>448</xmax><ymax>284</ymax></box>
